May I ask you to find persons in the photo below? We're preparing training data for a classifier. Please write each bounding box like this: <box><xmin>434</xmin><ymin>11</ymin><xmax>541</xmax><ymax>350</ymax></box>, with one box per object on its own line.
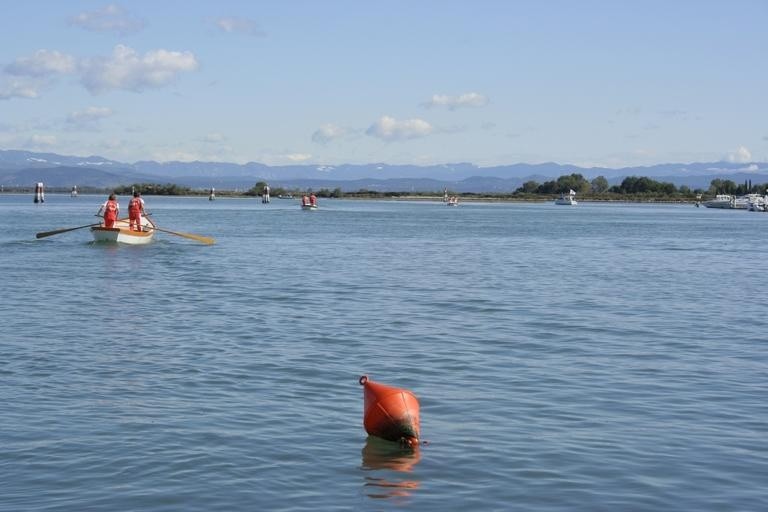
<box><xmin>127</xmin><ymin>191</ymin><xmax>147</xmax><ymax>233</ymax></box>
<box><xmin>303</xmin><ymin>195</ymin><xmax>307</xmax><ymax>204</ymax></box>
<box><xmin>310</xmin><ymin>192</ymin><xmax>316</xmax><ymax>205</ymax></box>
<box><xmin>97</xmin><ymin>192</ymin><xmax>119</xmax><ymax>228</ymax></box>
<box><xmin>448</xmin><ymin>194</ymin><xmax>457</xmax><ymax>203</ymax></box>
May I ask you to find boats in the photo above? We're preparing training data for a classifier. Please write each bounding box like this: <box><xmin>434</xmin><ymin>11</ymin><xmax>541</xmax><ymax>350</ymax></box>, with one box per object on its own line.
<box><xmin>91</xmin><ymin>215</ymin><xmax>156</xmax><ymax>244</ymax></box>
<box><xmin>701</xmin><ymin>193</ymin><xmax>768</xmax><ymax>211</ymax></box>
<box><xmin>301</xmin><ymin>204</ymin><xmax>318</xmax><ymax>210</ymax></box>
<box><xmin>555</xmin><ymin>196</ymin><xmax>578</xmax><ymax>205</ymax></box>
<box><xmin>447</xmin><ymin>203</ymin><xmax>458</xmax><ymax>206</ymax></box>
<box><xmin>279</xmin><ymin>195</ymin><xmax>296</xmax><ymax>199</ymax></box>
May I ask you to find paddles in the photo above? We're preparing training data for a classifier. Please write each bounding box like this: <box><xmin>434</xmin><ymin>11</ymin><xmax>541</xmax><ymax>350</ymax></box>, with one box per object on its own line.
<box><xmin>36</xmin><ymin>213</ymin><xmax>152</xmax><ymax>238</ymax></box>
<box><xmin>94</xmin><ymin>215</ymin><xmax>215</xmax><ymax>244</ymax></box>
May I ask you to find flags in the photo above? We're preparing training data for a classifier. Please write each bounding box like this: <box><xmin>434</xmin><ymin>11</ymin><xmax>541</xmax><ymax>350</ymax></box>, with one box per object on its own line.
<box><xmin>570</xmin><ymin>188</ymin><xmax>577</xmax><ymax>195</ymax></box>
